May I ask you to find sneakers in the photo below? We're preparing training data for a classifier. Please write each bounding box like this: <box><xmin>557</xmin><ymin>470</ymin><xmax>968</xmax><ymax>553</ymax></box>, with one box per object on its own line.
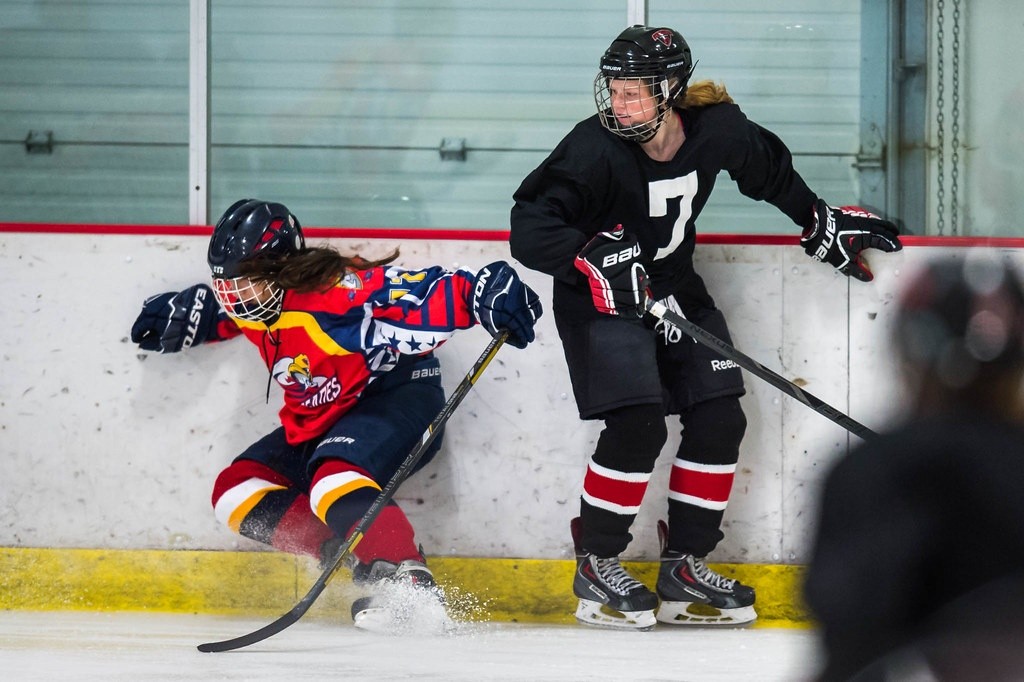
<box><xmin>352</xmin><ymin>543</ymin><xmax>455</xmax><ymax>633</ymax></box>
<box><xmin>655</xmin><ymin>519</ymin><xmax>758</xmax><ymax>627</ymax></box>
<box><xmin>317</xmin><ymin>538</ymin><xmax>373</xmax><ymax>585</ymax></box>
<box><xmin>571</xmin><ymin>517</ymin><xmax>658</xmax><ymax>628</ymax></box>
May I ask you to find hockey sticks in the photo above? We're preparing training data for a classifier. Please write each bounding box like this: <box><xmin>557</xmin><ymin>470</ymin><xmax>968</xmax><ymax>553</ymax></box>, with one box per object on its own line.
<box><xmin>194</xmin><ymin>327</ymin><xmax>512</xmax><ymax>656</ymax></box>
<box><xmin>647</xmin><ymin>296</ymin><xmax>880</xmax><ymax>443</ymax></box>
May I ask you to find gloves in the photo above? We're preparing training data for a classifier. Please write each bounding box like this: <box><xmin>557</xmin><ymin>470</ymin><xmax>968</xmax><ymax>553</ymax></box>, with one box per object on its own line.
<box><xmin>130</xmin><ymin>283</ymin><xmax>220</xmax><ymax>354</ymax></box>
<box><xmin>468</xmin><ymin>261</ymin><xmax>543</xmax><ymax>349</ymax></box>
<box><xmin>575</xmin><ymin>224</ymin><xmax>652</xmax><ymax>321</ymax></box>
<box><xmin>798</xmin><ymin>197</ymin><xmax>904</xmax><ymax>282</ymax></box>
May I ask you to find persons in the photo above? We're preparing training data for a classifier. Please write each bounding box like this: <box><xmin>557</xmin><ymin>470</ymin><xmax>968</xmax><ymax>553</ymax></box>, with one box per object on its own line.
<box><xmin>801</xmin><ymin>253</ymin><xmax>1024</xmax><ymax>682</ymax></box>
<box><xmin>132</xmin><ymin>199</ymin><xmax>543</xmax><ymax>634</ymax></box>
<box><xmin>509</xmin><ymin>25</ymin><xmax>902</xmax><ymax>632</ymax></box>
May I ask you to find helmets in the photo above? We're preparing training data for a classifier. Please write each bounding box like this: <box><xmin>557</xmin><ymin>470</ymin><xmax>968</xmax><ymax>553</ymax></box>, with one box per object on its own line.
<box><xmin>207</xmin><ymin>199</ymin><xmax>306</xmax><ymax>322</ymax></box>
<box><xmin>594</xmin><ymin>25</ymin><xmax>693</xmax><ymax>145</ymax></box>
